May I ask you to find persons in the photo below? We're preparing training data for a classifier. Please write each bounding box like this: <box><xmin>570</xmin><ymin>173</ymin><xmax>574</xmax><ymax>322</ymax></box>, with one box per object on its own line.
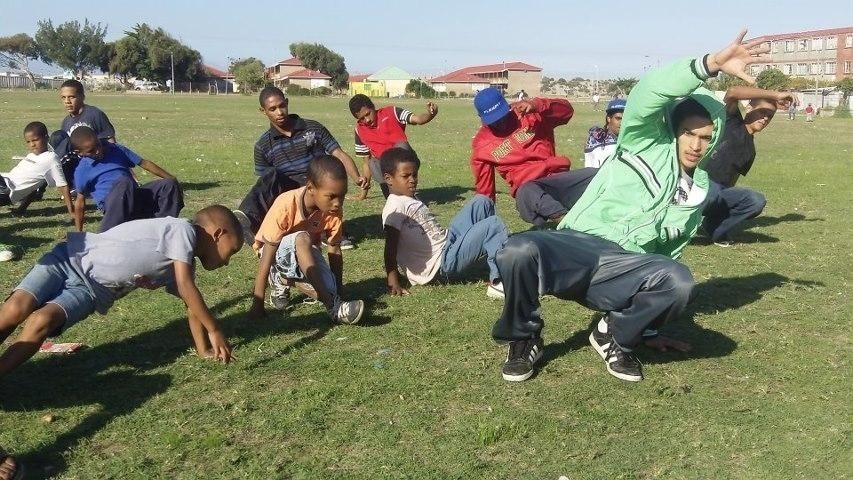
<box><xmin>380</xmin><ymin>26</ymin><xmax>815</xmax><ymax>385</ymax></box>
<box><xmin>0</xmin><ymin>86</ymin><xmax>437</xmax><ymax>380</ymax></box>
<box><xmin>1</xmin><ymin>79</ymin><xmax>183</xmax><ymax>233</ymax></box>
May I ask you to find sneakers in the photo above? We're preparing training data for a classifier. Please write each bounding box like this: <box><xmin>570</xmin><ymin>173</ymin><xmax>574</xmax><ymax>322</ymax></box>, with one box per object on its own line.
<box><xmin>502</xmin><ymin>337</ymin><xmax>544</xmax><ymax>381</ymax></box>
<box><xmin>713</xmin><ymin>239</ymin><xmax>735</xmax><ymax>248</ymax></box>
<box><xmin>589</xmin><ymin>324</ymin><xmax>644</xmax><ymax>382</ymax></box>
<box><xmin>486</xmin><ymin>281</ymin><xmax>505</xmax><ymax>302</ymax></box>
<box><xmin>332</xmin><ymin>299</ymin><xmax>364</xmax><ymax>325</ymax></box>
<box><xmin>233</xmin><ymin>209</ymin><xmax>255</xmax><ymax>248</ymax></box>
<box><xmin>268</xmin><ymin>270</ymin><xmax>292</xmax><ymax>311</ymax></box>
<box><xmin>322</xmin><ymin>233</ymin><xmax>352</xmax><ymax>251</ymax></box>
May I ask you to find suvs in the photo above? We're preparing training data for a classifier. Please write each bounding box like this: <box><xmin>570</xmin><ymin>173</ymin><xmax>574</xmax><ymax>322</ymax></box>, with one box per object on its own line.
<box><xmin>135</xmin><ymin>81</ymin><xmax>159</xmax><ymax>91</ymax></box>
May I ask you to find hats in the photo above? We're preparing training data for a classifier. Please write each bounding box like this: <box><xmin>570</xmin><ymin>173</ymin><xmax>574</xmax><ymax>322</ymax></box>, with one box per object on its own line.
<box><xmin>606</xmin><ymin>100</ymin><xmax>626</xmax><ymax>113</ymax></box>
<box><xmin>475</xmin><ymin>88</ymin><xmax>510</xmax><ymax>126</ymax></box>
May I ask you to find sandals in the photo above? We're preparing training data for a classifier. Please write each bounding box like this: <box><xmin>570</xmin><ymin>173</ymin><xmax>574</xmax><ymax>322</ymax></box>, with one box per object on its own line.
<box><xmin>0</xmin><ymin>445</ymin><xmax>27</xmax><ymax>480</ymax></box>
